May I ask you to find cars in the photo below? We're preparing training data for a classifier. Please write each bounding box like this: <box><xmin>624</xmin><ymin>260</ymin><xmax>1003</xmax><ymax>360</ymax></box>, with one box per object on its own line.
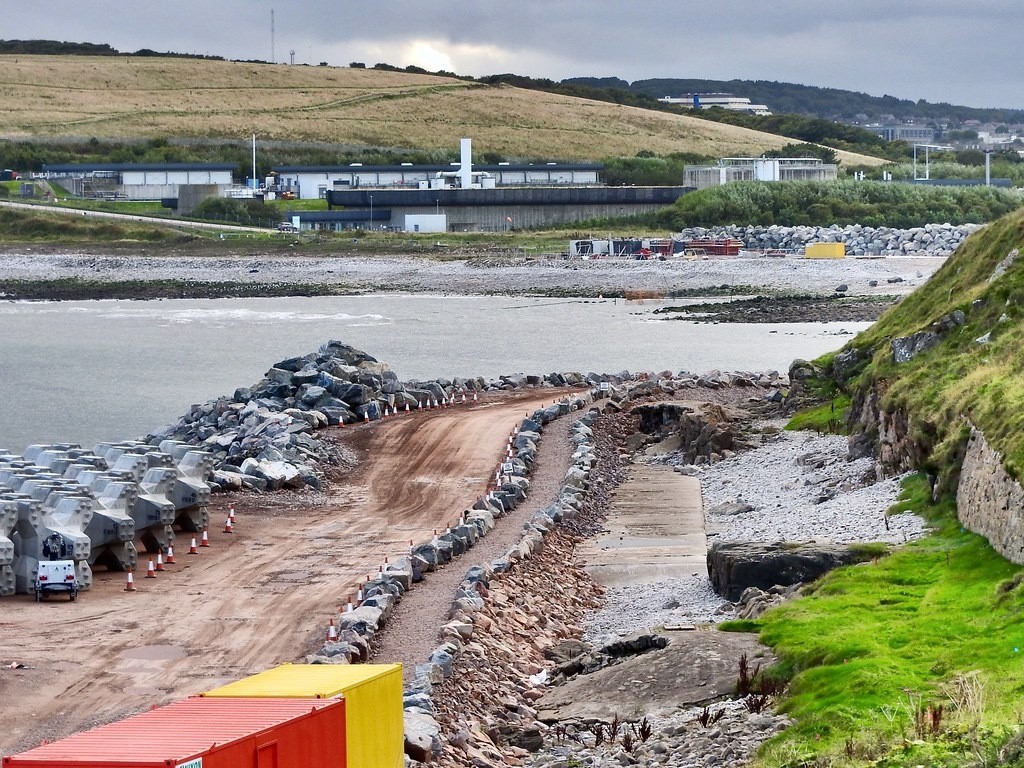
<box><xmin>278</xmin><ymin>222</ymin><xmax>298</xmax><ymax>231</ymax></box>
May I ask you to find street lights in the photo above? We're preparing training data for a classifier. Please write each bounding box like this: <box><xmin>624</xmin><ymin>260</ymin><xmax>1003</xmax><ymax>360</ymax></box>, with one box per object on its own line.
<box><xmin>370</xmin><ymin>195</ymin><xmax>373</xmax><ymax>229</ymax></box>
<box><xmin>436</xmin><ymin>199</ymin><xmax>440</xmax><ymax>215</ymax></box>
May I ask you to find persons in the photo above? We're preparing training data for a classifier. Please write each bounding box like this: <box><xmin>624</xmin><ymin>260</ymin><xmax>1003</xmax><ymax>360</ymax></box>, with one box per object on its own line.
<box><xmin>659</xmin><ymin>254</ymin><xmax>667</xmax><ymax>261</ymax></box>
<box><xmin>703</xmin><ymin>253</ymin><xmax>710</xmax><ymax>260</ymax></box>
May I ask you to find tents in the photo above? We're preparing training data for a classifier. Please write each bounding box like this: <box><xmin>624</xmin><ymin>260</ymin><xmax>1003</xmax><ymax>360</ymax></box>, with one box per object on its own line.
<box><xmin>629</xmin><ymin>249</ymin><xmax>650</xmax><ymax>260</ymax></box>
